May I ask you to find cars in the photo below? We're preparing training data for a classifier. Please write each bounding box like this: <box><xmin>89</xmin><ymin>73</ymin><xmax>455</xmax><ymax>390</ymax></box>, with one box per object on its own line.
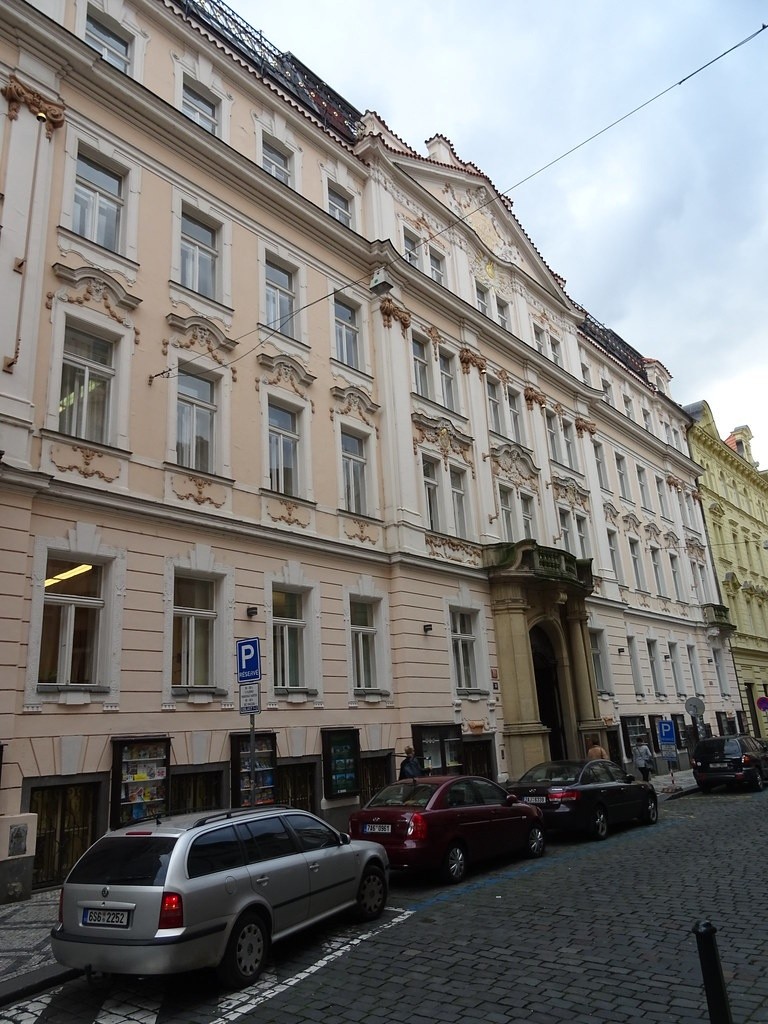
<box><xmin>510</xmin><ymin>759</ymin><xmax>657</xmax><ymax>842</ymax></box>
<box><xmin>350</xmin><ymin>773</ymin><xmax>543</xmax><ymax>881</ymax></box>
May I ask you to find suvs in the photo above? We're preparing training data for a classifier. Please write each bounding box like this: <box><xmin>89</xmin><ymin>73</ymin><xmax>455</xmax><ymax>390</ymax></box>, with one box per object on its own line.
<box><xmin>693</xmin><ymin>733</ymin><xmax>768</xmax><ymax>789</ymax></box>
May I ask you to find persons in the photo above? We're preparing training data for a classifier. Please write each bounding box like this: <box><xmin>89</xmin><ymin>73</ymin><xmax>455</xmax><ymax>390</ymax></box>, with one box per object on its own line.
<box><xmin>397</xmin><ymin>745</ymin><xmax>432</xmax><ymax>780</ymax></box>
<box><xmin>587</xmin><ymin>738</ymin><xmax>609</xmax><ymax>776</ymax></box>
<box><xmin>631</xmin><ymin>737</ymin><xmax>657</xmax><ymax>783</ymax></box>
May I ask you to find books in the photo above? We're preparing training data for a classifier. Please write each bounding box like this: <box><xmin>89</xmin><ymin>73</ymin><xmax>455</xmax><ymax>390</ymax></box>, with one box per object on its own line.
<box><xmin>120</xmin><ymin>744</ymin><xmax>167</xmax><ymax>822</ymax></box>
<box><xmin>240</xmin><ymin>739</ymin><xmax>274</xmax><ymax>807</ymax></box>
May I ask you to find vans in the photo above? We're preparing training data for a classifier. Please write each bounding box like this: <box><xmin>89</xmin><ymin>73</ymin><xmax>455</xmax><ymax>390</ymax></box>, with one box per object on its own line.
<box><xmin>50</xmin><ymin>809</ymin><xmax>391</xmax><ymax>993</ymax></box>
